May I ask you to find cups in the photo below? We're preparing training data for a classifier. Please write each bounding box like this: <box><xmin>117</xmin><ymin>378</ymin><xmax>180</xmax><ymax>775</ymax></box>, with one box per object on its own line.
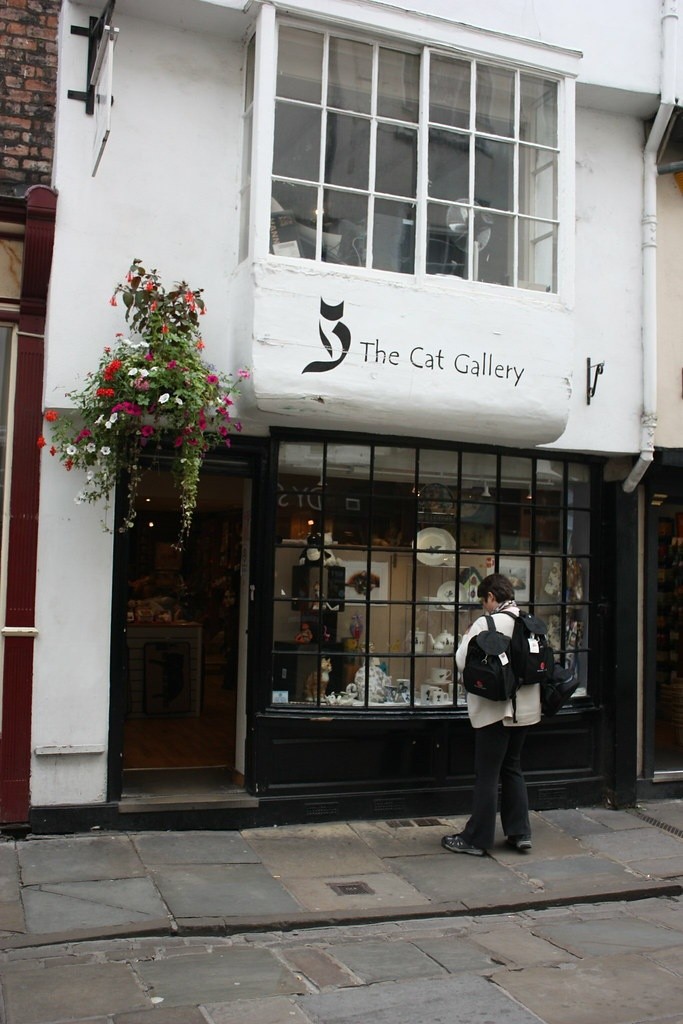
<box><xmin>432</xmin><ymin>692</ymin><xmax>448</xmax><ymax>704</ymax></box>
<box><xmin>430</xmin><ymin>687</ymin><xmax>442</xmax><ymax>704</ymax></box>
<box><xmin>421</xmin><ymin>686</ymin><xmax>430</xmax><ymax>704</ymax></box>
<box><xmin>384</xmin><ymin>685</ymin><xmax>396</xmax><ymax>702</ymax></box>
<box><xmin>397</xmin><ymin>679</ymin><xmax>410</xmax><ymax>703</ymax></box>
<box><xmin>407</xmin><ymin>628</ymin><xmax>425</xmax><ymax>652</ymax></box>
<box><xmin>431</xmin><ymin>668</ymin><xmax>451</xmax><ymax>682</ymax></box>
<box><xmin>448</xmin><ymin>684</ymin><xmax>462</xmax><ymax>701</ymax></box>
<box><xmin>431</xmin><ymin>641</ymin><xmax>443</xmax><ymax>652</ymax></box>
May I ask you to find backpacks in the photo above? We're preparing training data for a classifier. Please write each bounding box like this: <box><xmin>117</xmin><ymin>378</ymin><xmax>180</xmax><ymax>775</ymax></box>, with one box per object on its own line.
<box><xmin>492</xmin><ymin>607</ymin><xmax>556</xmax><ymax>684</ymax></box>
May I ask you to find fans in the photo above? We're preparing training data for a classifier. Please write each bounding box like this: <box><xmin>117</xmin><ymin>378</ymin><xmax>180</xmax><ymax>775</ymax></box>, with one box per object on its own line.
<box><xmin>443</xmin><ymin>198</ymin><xmax>495</xmax><ymax>281</ymax></box>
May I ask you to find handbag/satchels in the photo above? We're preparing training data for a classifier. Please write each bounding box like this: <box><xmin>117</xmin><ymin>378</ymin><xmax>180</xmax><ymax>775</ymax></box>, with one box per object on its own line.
<box><xmin>462</xmin><ymin>614</ymin><xmax>526</xmax><ymax>724</ymax></box>
<box><xmin>539</xmin><ymin>659</ymin><xmax>580</xmax><ymax>716</ymax></box>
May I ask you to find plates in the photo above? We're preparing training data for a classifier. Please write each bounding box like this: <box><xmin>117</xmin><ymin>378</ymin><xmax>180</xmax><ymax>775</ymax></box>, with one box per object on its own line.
<box><xmin>425</xmin><ymin>680</ymin><xmax>451</xmax><ymax>685</ymax></box>
<box><xmin>437</xmin><ymin>581</ymin><xmax>468</xmax><ymax>610</ymax></box>
<box><xmin>412</xmin><ymin>527</ymin><xmax>456</xmax><ymax>566</ymax></box>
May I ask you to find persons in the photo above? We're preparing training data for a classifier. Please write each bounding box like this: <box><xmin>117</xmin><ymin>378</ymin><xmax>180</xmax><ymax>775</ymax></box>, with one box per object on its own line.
<box><xmin>442</xmin><ymin>574</ymin><xmax>579</xmax><ymax>856</ymax></box>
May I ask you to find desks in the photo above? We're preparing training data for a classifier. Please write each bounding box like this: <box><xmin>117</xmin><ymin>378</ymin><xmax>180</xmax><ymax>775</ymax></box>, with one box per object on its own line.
<box><xmin>426</xmin><ymin>224</ymin><xmax>467</xmax><ymax>279</ymax></box>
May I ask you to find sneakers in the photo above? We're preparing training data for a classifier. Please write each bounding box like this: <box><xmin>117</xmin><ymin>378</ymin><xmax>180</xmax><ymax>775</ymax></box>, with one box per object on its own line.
<box><xmin>441</xmin><ymin>831</ymin><xmax>487</xmax><ymax>856</ymax></box>
<box><xmin>507</xmin><ymin>835</ymin><xmax>532</xmax><ymax>849</ymax></box>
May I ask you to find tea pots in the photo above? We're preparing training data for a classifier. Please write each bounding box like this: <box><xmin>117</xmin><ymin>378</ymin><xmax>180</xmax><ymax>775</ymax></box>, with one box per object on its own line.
<box><xmin>429</xmin><ymin>629</ymin><xmax>463</xmax><ymax>652</ymax></box>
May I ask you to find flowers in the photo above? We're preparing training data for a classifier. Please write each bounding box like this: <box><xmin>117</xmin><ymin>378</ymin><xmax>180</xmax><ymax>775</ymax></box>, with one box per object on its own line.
<box><xmin>36</xmin><ymin>260</ymin><xmax>254</xmax><ymax>554</ymax></box>
